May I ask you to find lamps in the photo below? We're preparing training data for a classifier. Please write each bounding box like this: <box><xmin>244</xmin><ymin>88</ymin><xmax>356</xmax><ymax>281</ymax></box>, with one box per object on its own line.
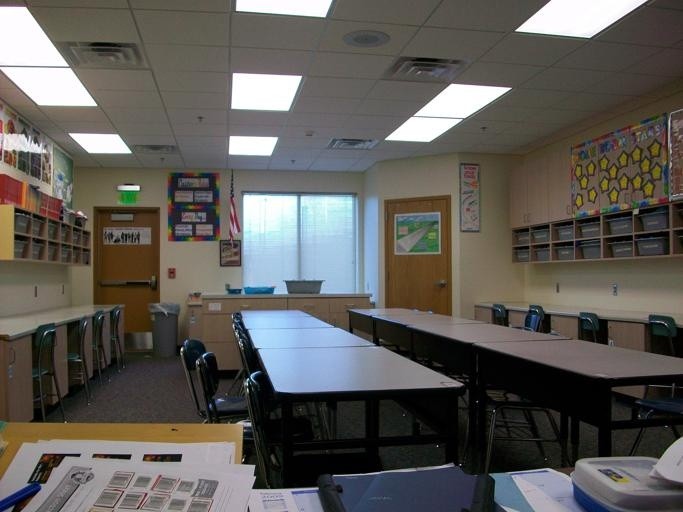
<box><xmin>0</xmin><ymin>302</ymin><xmax>127</xmax><ymax>423</ymax></box>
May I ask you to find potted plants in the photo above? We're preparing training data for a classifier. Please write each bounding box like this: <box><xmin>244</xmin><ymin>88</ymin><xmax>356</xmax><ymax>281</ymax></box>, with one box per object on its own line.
<box><xmin>217</xmin><ymin>238</ymin><xmax>241</xmax><ymax>267</ymax></box>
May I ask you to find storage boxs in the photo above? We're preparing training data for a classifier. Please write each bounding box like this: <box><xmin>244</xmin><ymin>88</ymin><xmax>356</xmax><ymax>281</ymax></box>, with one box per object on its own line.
<box><xmin>0</xmin><ymin>173</ymin><xmax>92</xmax><ymax>266</ymax></box>
<box><xmin>568</xmin><ymin>453</ymin><xmax>682</xmax><ymax>512</ymax></box>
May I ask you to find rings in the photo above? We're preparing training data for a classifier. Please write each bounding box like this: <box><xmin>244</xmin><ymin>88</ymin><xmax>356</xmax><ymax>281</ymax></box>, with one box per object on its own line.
<box><xmin>0</xmin><ymin>482</ymin><xmax>41</xmax><ymax>512</ymax></box>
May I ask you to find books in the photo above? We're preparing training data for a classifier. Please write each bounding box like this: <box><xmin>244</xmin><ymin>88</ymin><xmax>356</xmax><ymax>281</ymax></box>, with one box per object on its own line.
<box><xmin>0</xmin><ymin>173</ymin><xmax>92</xmax><ymax>266</ymax></box>
<box><xmin>568</xmin><ymin>453</ymin><xmax>682</xmax><ymax>512</ymax></box>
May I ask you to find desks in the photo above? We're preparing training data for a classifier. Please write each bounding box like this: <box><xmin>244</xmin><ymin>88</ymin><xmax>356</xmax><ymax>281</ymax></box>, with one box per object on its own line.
<box><xmin>0</xmin><ymin>412</ymin><xmax>243</xmax><ymax>512</ymax></box>
<box><xmin>249</xmin><ymin>462</ymin><xmax>683</xmax><ymax>511</ymax></box>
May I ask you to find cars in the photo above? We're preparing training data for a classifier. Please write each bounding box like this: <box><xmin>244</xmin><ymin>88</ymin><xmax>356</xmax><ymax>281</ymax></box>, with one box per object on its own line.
<box><xmin>192</xmin><ymin>292</ymin><xmax>201</xmax><ymax>297</ymax></box>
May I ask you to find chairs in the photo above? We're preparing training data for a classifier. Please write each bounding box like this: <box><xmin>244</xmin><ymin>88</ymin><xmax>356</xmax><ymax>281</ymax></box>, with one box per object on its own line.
<box><xmin>0</xmin><ymin>302</ymin><xmax>127</xmax><ymax>423</ymax></box>
<box><xmin>233</xmin><ymin>303</ymin><xmax>683</xmax><ymax>486</ymax></box>
<box><xmin>180</xmin><ymin>339</ymin><xmax>210</xmax><ymax>423</ymax></box>
<box><xmin>196</xmin><ymin>352</ymin><xmax>248</xmax><ymax>423</ymax></box>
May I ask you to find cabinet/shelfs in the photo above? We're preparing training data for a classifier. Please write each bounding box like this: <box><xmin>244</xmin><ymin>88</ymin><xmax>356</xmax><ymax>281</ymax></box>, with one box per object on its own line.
<box><xmin>55</xmin><ymin>176</ymin><xmax>62</xmax><ymax>199</ymax></box>
<box><xmin>503</xmin><ymin>76</ymin><xmax>683</xmax><ymax>263</ymax></box>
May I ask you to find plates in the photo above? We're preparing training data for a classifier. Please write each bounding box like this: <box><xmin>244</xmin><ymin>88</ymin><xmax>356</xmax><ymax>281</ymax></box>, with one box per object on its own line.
<box><xmin>503</xmin><ymin>76</ymin><xmax>683</xmax><ymax>263</ymax></box>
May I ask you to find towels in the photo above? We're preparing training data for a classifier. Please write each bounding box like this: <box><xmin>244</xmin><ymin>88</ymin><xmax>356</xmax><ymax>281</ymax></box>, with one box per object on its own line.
<box><xmin>148</xmin><ymin>302</ymin><xmax>179</xmax><ymax>359</ymax></box>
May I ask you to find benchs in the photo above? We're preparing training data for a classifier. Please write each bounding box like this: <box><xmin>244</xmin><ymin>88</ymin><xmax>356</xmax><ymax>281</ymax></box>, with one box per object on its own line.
<box><xmin>229</xmin><ymin>171</ymin><xmax>241</xmax><ymax>241</ymax></box>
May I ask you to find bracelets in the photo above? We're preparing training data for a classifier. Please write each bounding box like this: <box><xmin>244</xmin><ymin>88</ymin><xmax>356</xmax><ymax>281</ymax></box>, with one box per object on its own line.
<box><xmin>225</xmin><ymin>288</ymin><xmax>241</xmax><ymax>294</ymax></box>
<box><xmin>242</xmin><ymin>287</ymin><xmax>274</xmax><ymax>294</ymax></box>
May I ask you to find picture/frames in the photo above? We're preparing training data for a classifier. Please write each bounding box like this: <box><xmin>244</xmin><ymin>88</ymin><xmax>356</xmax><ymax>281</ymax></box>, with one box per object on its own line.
<box><xmin>249</xmin><ymin>462</ymin><xmax>683</xmax><ymax>511</ymax></box>
<box><xmin>0</xmin><ymin>412</ymin><xmax>243</xmax><ymax>512</ymax></box>
<box><xmin>217</xmin><ymin>238</ymin><xmax>241</xmax><ymax>267</ymax></box>
